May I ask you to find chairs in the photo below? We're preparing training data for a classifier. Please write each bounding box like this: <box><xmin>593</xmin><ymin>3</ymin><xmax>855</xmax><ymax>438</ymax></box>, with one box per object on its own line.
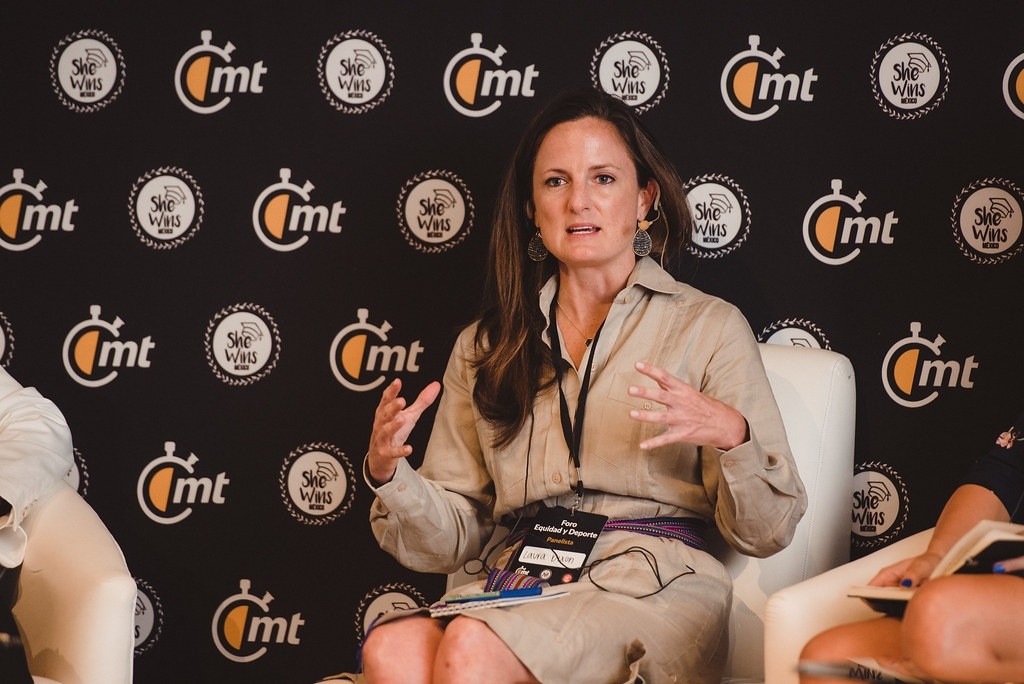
<box><xmin>714</xmin><ymin>344</ymin><xmax>856</xmax><ymax>683</ymax></box>
<box><xmin>764</xmin><ymin>525</ymin><xmax>936</xmax><ymax>683</ymax></box>
<box><xmin>11</xmin><ymin>479</ymin><xmax>137</xmax><ymax>684</ymax></box>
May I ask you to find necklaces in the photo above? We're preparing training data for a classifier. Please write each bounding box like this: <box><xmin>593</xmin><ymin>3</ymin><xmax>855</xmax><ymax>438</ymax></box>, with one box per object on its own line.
<box><xmin>555</xmin><ymin>300</ymin><xmax>594</xmax><ymax>348</ymax></box>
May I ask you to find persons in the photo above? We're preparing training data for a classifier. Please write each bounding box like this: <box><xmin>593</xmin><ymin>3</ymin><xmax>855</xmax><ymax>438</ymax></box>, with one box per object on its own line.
<box><xmin>0</xmin><ymin>363</ymin><xmax>79</xmax><ymax>684</ymax></box>
<box><xmin>798</xmin><ymin>388</ymin><xmax>1024</xmax><ymax>683</ymax></box>
<box><xmin>359</xmin><ymin>92</ymin><xmax>807</xmax><ymax>684</ymax></box>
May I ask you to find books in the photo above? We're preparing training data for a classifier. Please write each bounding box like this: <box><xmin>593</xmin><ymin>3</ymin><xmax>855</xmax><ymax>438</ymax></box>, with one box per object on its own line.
<box><xmin>846</xmin><ymin>516</ymin><xmax>1024</xmax><ymax>615</ymax></box>
